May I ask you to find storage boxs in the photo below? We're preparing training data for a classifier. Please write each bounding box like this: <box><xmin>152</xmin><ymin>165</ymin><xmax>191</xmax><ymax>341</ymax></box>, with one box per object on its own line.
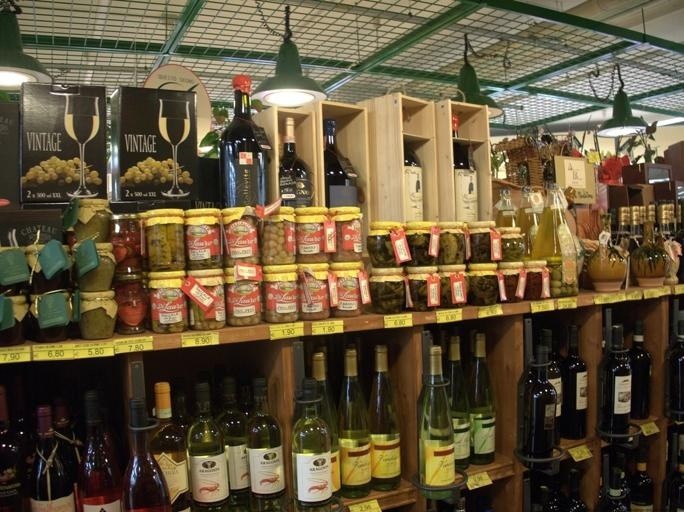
<box><xmin>0</xmin><ymin>203</ymin><xmax>70</xmax><ymax>290</ymax></box>
<box><xmin>0</xmin><ymin>86</ymin><xmax>199</xmax><ymax>201</ymax></box>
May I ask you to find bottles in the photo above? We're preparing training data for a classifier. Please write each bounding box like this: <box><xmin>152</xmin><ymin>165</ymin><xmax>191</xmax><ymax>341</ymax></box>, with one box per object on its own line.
<box><xmin>0</xmin><ymin>316</ymin><xmax>684</xmax><ymax>512</ymax></box>
<box><xmin>275</xmin><ymin>114</ymin><xmax>314</xmax><ymax>209</ymax></box>
<box><xmin>322</xmin><ymin>115</ymin><xmax>359</xmax><ymax>207</ymax></box>
<box><xmin>451</xmin><ymin>111</ymin><xmax>481</xmax><ymax>223</ymax></box>
<box><xmin>400</xmin><ymin>133</ymin><xmax>426</xmax><ymax>223</ymax></box>
<box><xmin>491</xmin><ymin>178</ymin><xmax>680</xmax><ymax>291</ymax></box>
<box><xmin>219</xmin><ymin>71</ymin><xmax>269</xmax><ymax>209</ymax></box>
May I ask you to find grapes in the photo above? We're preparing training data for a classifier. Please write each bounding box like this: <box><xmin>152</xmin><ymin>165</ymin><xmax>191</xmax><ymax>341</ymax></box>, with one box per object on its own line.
<box><xmin>120</xmin><ymin>156</ymin><xmax>193</xmax><ymax>188</ymax></box>
<box><xmin>22</xmin><ymin>155</ymin><xmax>102</xmax><ymax>187</ymax></box>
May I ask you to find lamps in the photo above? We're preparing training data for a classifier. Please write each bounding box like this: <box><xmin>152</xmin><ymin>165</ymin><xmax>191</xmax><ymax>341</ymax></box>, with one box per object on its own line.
<box><xmin>250</xmin><ymin>6</ymin><xmax>329</xmax><ymax>109</ymax></box>
<box><xmin>451</xmin><ymin>34</ymin><xmax>504</xmax><ymax>119</ymax></box>
<box><xmin>0</xmin><ymin>0</ymin><xmax>54</xmax><ymax>93</ymax></box>
<box><xmin>596</xmin><ymin>64</ymin><xmax>650</xmax><ymax>137</ymax></box>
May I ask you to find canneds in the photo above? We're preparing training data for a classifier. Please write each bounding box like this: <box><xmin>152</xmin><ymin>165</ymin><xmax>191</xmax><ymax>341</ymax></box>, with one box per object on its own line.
<box><xmin>139</xmin><ymin>206</ymin><xmax>370</xmax><ymax>334</ymax></box>
<box><xmin>0</xmin><ymin>199</ymin><xmax>146</xmax><ymax>348</ymax></box>
<box><xmin>367</xmin><ymin>221</ymin><xmax>579</xmax><ymax>314</ymax></box>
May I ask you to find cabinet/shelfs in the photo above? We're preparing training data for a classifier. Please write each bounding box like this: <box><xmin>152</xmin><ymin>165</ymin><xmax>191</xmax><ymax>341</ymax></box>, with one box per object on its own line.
<box><xmin>252</xmin><ymin>106</ymin><xmax>319</xmax><ymax>207</ymax></box>
<box><xmin>436</xmin><ymin>99</ymin><xmax>493</xmax><ymax>221</ymax></box>
<box><xmin>0</xmin><ymin>283</ymin><xmax>684</xmax><ymax>511</ymax></box>
<box><xmin>297</xmin><ymin>100</ymin><xmax>372</xmax><ymax>231</ymax></box>
<box><xmin>357</xmin><ymin>92</ymin><xmax>439</xmax><ymax>224</ymax></box>
